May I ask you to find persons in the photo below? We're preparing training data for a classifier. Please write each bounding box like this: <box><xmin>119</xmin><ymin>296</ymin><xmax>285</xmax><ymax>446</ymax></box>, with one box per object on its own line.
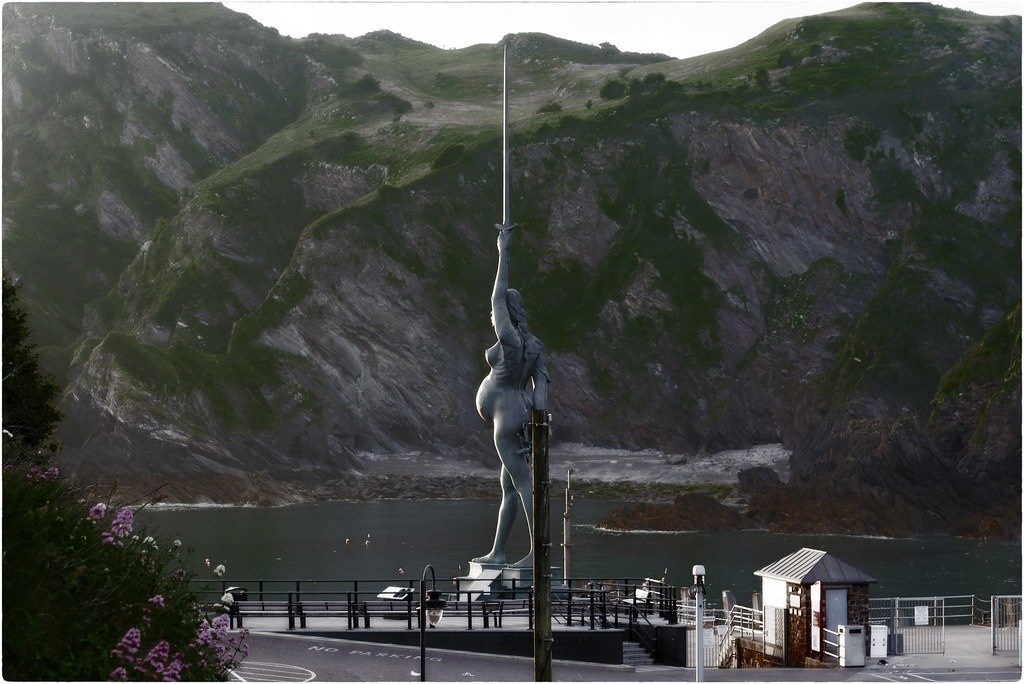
<box><xmin>470</xmin><ymin>229</ymin><xmax>551</xmax><ymax>568</ymax></box>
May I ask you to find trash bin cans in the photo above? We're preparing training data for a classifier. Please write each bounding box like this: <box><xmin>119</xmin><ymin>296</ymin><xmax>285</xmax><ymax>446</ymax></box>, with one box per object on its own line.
<box><xmin>837</xmin><ymin>625</ymin><xmax>865</xmax><ymax>667</ymax></box>
<box><xmin>225</xmin><ymin>586</ymin><xmax>247</xmax><ymax>617</ymax></box>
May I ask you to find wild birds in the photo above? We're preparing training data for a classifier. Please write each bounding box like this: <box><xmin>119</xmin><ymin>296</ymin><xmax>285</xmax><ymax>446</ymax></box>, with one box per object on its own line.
<box><xmin>367</xmin><ymin>533</ymin><xmax>371</xmax><ymax>537</ymax></box>
<box><xmin>345</xmin><ymin>538</ymin><xmax>350</xmax><ymax>542</ymax></box>
<box><xmin>364</xmin><ymin>539</ymin><xmax>371</xmax><ymax>545</ymax></box>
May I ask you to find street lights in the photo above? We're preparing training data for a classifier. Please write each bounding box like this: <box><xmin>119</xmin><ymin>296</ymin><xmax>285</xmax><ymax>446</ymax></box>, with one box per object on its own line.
<box><xmin>420</xmin><ymin>563</ymin><xmax>446</xmax><ymax>682</ymax></box>
<box><xmin>692</xmin><ymin>564</ymin><xmax>706</xmax><ymax>682</ymax></box>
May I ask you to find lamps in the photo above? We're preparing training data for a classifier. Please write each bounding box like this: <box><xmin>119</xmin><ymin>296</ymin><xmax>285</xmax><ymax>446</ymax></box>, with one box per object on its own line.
<box><xmin>420</xmin><ymin>564</ymin><xmax>447</xmax><ymax>681</ymax></box>
